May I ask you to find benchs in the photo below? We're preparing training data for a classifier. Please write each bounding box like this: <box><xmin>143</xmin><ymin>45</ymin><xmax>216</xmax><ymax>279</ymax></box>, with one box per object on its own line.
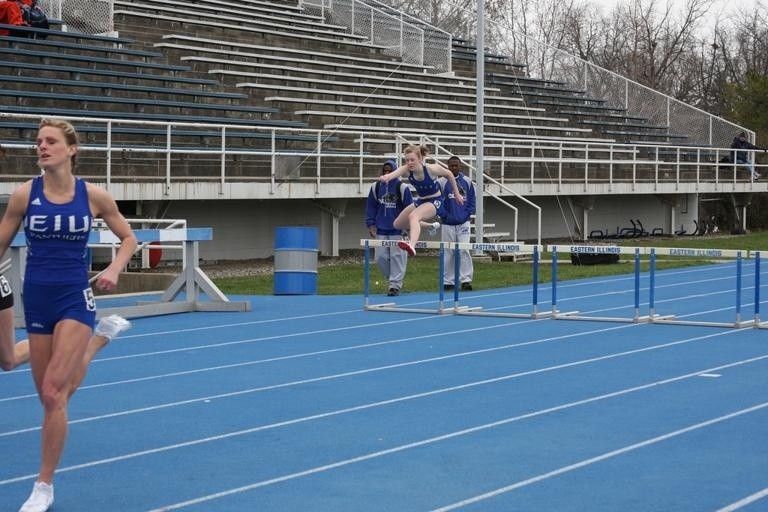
<box><xmin>154</xmin><ymin>35</ymin><xmax>618</xmax><ymax>185</ymax></box>
<box><xmin>1</xmin><ymin>20</ymin><xmax>338</xmax><ymax>184</ymax></box>
<box><xmin>113</xmin><ymin>1</ymin><xmax>390</xmax><ymax>54</ymax></box>
<box><xmin>484</xmin><ymin>72</ymin><xmax>747</xmax><ymax>183</ymax></box>
<box><xmin>335</xmin><ymin>0</ymin><xmax>528</xmax><ymax>75</ymax></box>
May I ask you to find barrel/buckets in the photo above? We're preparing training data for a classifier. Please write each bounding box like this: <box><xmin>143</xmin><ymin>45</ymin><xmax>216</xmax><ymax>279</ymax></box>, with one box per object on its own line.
<box><xmin>274</xmin><ymin>226</ymin><xmax>319</xmax><ymax>295</ymax></box>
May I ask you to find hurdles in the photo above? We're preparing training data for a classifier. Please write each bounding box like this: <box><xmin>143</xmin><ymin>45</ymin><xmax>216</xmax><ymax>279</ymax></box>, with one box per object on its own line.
<box><xmin>749</xmin><ymin>250</ymin><xmax>768</xmax><ymax>329</ymax></box>
<box><xmin>644</xmin><ymin>246</ymin><xmax>761</xmax><ymax>329</ymax></box>
<box><xmin>361</xmin><ymin>238</ymin><xmax>469</xmax><ymax>316</ymax></box>
<box><xmin>449</xmin><ymin>241</ymin><xmax>560</xmax><ymax>320</ymax></box>
<box><xmin>546</xmin><ymin>244</ymin><xmax>660</xmax><ymax>323</ymax></box>
<box><xmin>10</xmin><ymin>228</ymin><xmax>250</xmax><ymax>329</ymax></box>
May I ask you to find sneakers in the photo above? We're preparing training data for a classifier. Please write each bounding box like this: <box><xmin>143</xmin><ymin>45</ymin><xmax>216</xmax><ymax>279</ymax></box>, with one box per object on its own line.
<box><xmin>444</xmin><ymin>282</ymin><xmax>454</xmax><ymax>290</ymax></box>
<box><xmin>427</xmin><ymin>222</ymin><xmax>441</xmax><ymax>238</ymax></box>
<box><xmin>397</xmin><ymin>240</ymin><xmax>417</xmax><ymax>257</ymax></box>
<box><xmin>386</xmin><ymin>287</ymin><xmax>400</xmax><ymax>296</ymax></box>
<box><xmin>461</xmin><ymin>282</ymin><xmax>473</xmax><ymax>291</ymax></box>
<box><xmin>94</xmin><ymin>313</ymin><xmax>133</xmax><ymax>344</ymax></box>
<box><xmin>18</xmin><ymin>480</ymin><xmax>55</xmax><ymax>512</ymax></box>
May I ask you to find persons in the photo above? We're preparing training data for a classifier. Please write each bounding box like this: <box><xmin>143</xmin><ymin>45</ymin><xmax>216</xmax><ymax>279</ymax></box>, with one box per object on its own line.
<box><xmin>0</xmin><ymin>274</ymin><xmax>30</xmax><ymax>371</ymax></box>
<box><xmin>366</xmin><ymin>160</ymin><xmax>413</xmax><ymax>296</ymax></box>
<box><xmin>0</xmin><ymin>0</ymin><xmax>41</xmax><ymax>35</ymax></box>
<box><xmin>0</xmin><ymin>118</ymin><xmax>138</xmax><ymax>512</ymax></box>
<box><xmin>731</xmin><ymin>132</ymin><xmax>767</xmax><ymax>180</ymax></box>
<box><xmin>437</xmin><ymin>156</ymin><xmax>476</xmax><ymax>291</ymax></box>
<box><xmin>378</xmin><ymin>144</ymin><xmax>464</xmax><ymax>256</ymax></box>
